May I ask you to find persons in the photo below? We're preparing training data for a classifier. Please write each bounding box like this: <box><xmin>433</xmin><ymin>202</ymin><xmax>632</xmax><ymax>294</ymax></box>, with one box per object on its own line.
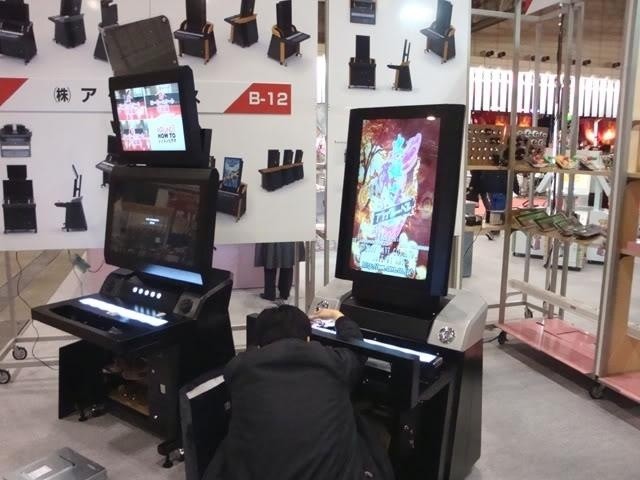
<box><xmin>202</xmin><ymin>303</ymin><xmax>394</xmax><ymax>479</ymax></box>
<box><xmin>479</xmin><ymin>170</ymin><xmax>519</xmax><ymax>240</ymax></box>
<box><xmin>254</xmin><ymin>241</ymin><xmax>306</xmax><ymax>301</ymax></box>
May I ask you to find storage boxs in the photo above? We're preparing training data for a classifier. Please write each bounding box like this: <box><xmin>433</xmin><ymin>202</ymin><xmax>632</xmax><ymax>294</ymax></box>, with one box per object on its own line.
<box><xmin>1</xmin><ymin>442</ymin><xmax>113</xmax><ymax>479</ymax></box>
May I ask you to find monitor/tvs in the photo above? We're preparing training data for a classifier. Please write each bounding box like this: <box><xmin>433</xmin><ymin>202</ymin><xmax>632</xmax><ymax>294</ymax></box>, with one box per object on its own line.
<box><xmin>333</xmin><ymin>102</ymin><xmax>466</xmax><ymax>311</ymax></box>
<box><xmin>104</xmin><ymin>167</ymin><xmax>218</xmax><ymax>285</ymax></box>
<box><xmin>109</xmin><ymin>65</ymin><xmax>201</xmax><ymax>166</ymax></box>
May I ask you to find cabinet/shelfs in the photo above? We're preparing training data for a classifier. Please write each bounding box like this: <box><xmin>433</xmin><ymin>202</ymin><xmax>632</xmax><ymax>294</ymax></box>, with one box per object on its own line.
<box><xmin>467</xmin><ymin>161</ymin><xmax>537</xmax><ymax>326</ymax></box>
<box><xmin>497</xmin><ymin>158</ymin><xmax>619</xmax><ymax>400</ymax></box>
<box><xmin>591</xmin><ymin>106</ymin><xmax>639</xmax><ymax>408</ymax></box>
